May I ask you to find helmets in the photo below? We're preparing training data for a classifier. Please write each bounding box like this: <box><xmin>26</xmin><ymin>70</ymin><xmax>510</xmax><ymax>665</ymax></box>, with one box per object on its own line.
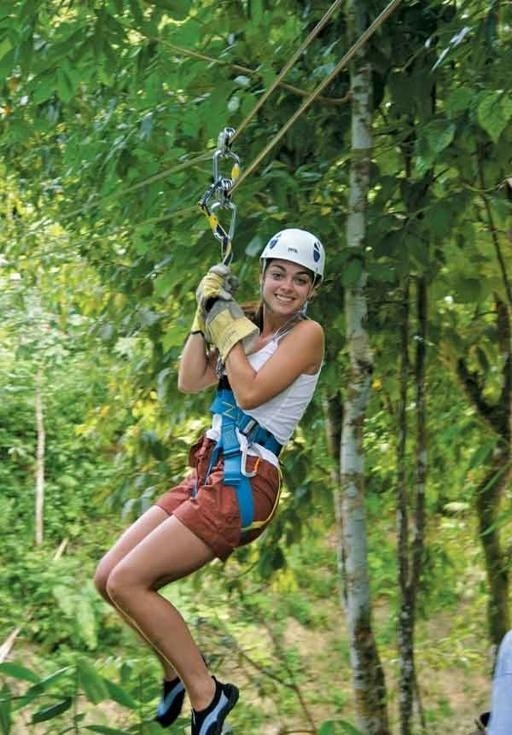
<box><xmin>259</xmin><ymin>228</ymin><xmax>326</xmax><ymax>297</ymax></box>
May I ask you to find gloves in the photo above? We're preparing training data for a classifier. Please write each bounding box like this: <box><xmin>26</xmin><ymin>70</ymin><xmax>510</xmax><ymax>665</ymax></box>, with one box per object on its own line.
<box><xmin>190</xmin><ymin>262</ymin><xmax>261</xmax><ymax>375</ymax></box>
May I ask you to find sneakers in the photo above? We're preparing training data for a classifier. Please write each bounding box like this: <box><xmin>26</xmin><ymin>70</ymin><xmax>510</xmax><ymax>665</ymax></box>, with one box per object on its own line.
<box><xmin>191</xmin><ymin>675</ymin><xmax>239</xmax><ymax>735</ymax></box>
<box><xmin>156</xmin><ymin>654</ymin><xmax>207</xmax><ymax>728</ymax></box>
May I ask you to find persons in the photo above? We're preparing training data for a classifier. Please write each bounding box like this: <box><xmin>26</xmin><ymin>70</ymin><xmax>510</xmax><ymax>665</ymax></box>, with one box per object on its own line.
<box><xmin>91</xmin><ymin>227</ymin><xmax>329</xmax><ymax>734</ymax></box>
